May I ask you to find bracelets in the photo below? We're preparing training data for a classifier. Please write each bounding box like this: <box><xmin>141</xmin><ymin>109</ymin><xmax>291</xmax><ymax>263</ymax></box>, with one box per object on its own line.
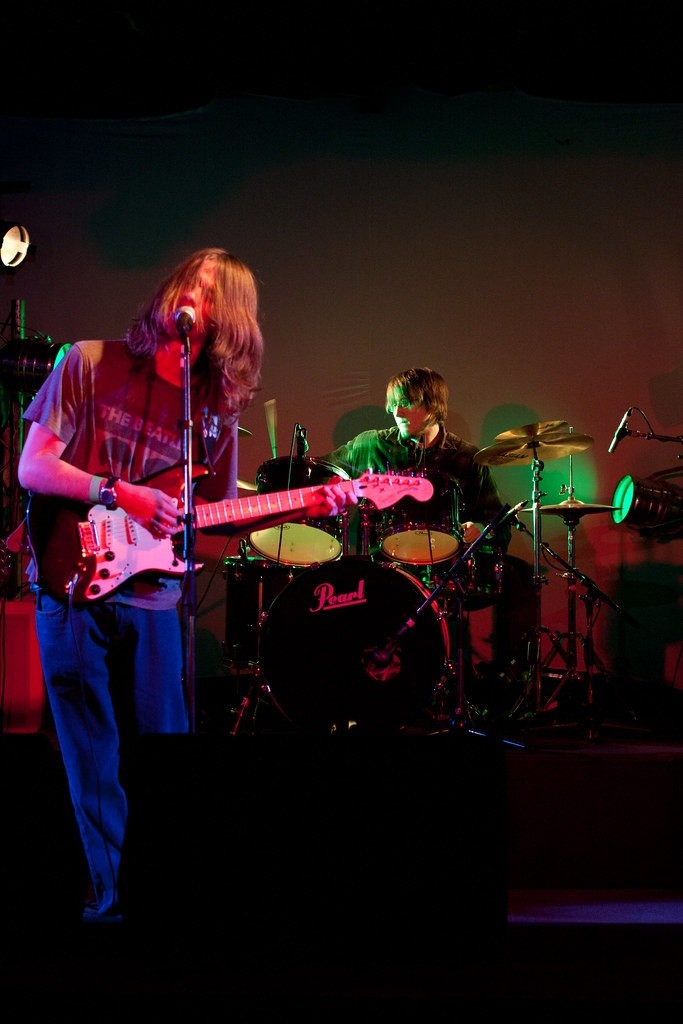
<box><xmin>89</xmin><ymin>476</ymin><xmax>103</xmax><ymax>504</ymax></box>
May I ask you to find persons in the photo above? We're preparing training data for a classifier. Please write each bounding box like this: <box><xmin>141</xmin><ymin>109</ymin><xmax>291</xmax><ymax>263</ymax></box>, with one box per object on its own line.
<box><xmin>319</xmin><ymin>367</ymin><xmax>540</xmax><ymax>669</ymax></box>
<box><xmin>17</xmin><ymin>246</ymin><xmax>360</xmax><ymax>925</ymax></box>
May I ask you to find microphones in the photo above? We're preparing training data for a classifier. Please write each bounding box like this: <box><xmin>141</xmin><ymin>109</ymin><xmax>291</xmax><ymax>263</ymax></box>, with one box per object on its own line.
<box><xmin>174</xmin><ymin>306</ymin><xmax>195</xmax><ymax>334</ymax></box>
<box><xmin>607</xmin><ymin>406</ymin><xmax>634</xmax><ymax>453</ymax></box>
<box><xmin>497</xmin><ymin>500</ymin><xmax>530</xmax><ymax>531</ymax></box>
<box><xmin>295</xmin><ymin>424</ymin><xmax>309</xmax><ymax>456</ymax></box>
<box><xmin>372</xmin><ymin>648</ymin><xmax>393</xmax><ymax>666</ymax></box>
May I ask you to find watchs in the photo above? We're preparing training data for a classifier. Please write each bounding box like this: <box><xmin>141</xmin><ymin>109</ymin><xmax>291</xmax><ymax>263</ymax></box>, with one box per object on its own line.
<box><xmin>100</xmin><ymin>476</ymin><xmax>122</xmax><ymax>511</ymax></box>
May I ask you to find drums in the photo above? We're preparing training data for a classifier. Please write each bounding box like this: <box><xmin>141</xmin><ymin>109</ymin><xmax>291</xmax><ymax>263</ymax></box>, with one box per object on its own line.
<box><xmin>421</xmin><ymin>542</ymin><xmax>509</xmax><ymax>600</ymax></box>
<box><xmin>375</xmin><ymin>466</ymin><xmax>466</xmax><ymax>565</ymax></box>
<box><xmin>255</xmin><ymin>553</ymin><xmax>448</xmax><ymax>728</ymax></box>
<box><xmin>250</xmin><ymin>455</ymin><xmax>351</xmax><ymax>568</ymax></box>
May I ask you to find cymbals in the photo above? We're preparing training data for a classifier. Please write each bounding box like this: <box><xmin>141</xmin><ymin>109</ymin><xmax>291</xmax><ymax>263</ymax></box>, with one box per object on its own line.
<box><xmin>472</xmin><ymin>431</ymin><xmax>594</xmax><ymax>467</ymax></box>
<box><xmin>493</xmin><ymin>419</ymin><xmax>567</xmax><ymax>441</ymax></box>
<box><xmin>520</xmin><ymin>498</ymin><xmax>623</xmax><ymax>515</ymax></box>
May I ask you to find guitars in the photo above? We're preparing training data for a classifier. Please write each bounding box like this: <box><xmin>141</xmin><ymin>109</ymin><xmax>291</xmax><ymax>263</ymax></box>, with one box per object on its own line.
<box><xmin>21</xmin><ymin>461</ymin><xmax>436</xmax><ymax>606</ymax></box>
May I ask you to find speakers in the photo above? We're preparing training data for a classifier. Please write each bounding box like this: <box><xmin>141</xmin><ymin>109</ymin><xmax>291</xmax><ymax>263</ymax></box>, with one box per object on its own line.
<box><xmin>121</xmin><ymin>736</ymin><xmax>514</xmax><ymax>989</ymax></box>
<box><xmin>0</xmin><ymin>731</ymin><xmax>90</xmax><ymax>1000</ymax></box>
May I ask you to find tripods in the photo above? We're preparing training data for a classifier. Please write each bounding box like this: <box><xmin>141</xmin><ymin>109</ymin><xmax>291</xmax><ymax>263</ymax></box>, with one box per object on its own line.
<box><xmin>424</xmin><ymin>510</ymin><xmax>642</xmax><ymax>750</ymax></box>
